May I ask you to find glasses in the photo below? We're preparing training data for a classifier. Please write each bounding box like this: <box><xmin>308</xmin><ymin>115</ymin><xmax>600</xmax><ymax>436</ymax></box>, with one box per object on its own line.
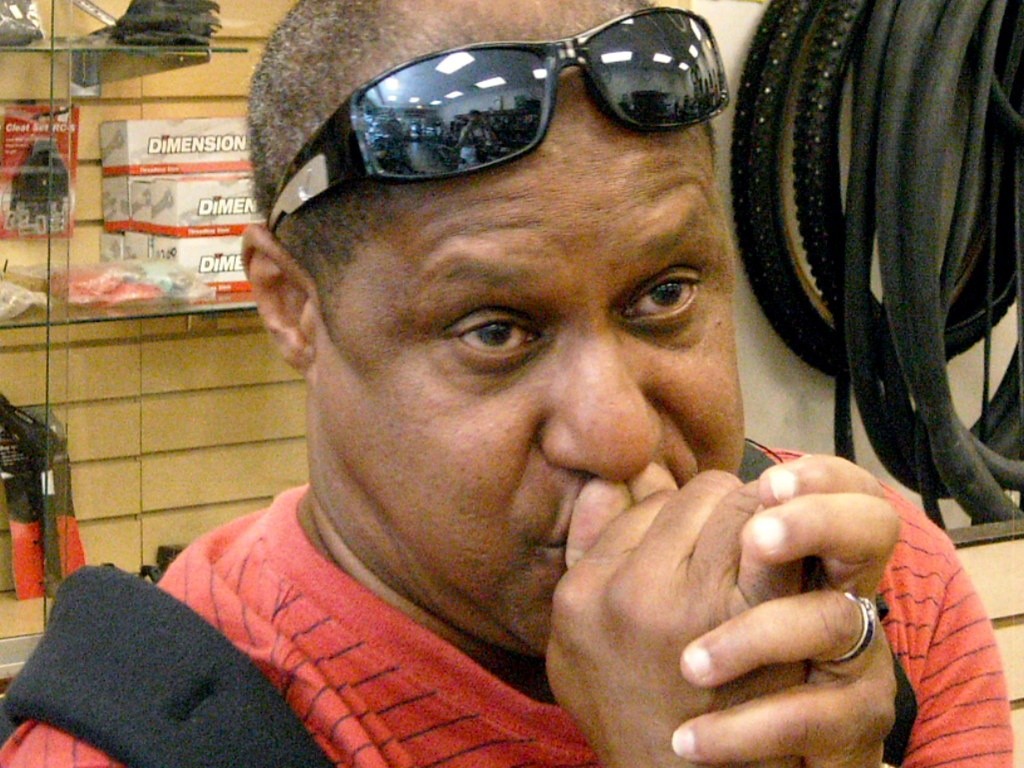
<box><xmin>265</xmin><ymin>6</ymin><xmax>730</xmax><ymax>237</ymax></box>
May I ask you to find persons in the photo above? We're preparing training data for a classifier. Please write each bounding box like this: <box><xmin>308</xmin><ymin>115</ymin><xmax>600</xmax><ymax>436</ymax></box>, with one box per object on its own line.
<box><xmin>0</xmin><ymin>0</ymin><xmax>1014</xmax><ymax>768</ymax></box>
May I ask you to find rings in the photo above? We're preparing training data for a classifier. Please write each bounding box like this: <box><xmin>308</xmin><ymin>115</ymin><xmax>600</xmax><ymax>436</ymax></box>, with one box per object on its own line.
<box><xmin>826</xmin><ymin>591</ymin><xmax>877</xmax><ymax>666</ymax></box>
